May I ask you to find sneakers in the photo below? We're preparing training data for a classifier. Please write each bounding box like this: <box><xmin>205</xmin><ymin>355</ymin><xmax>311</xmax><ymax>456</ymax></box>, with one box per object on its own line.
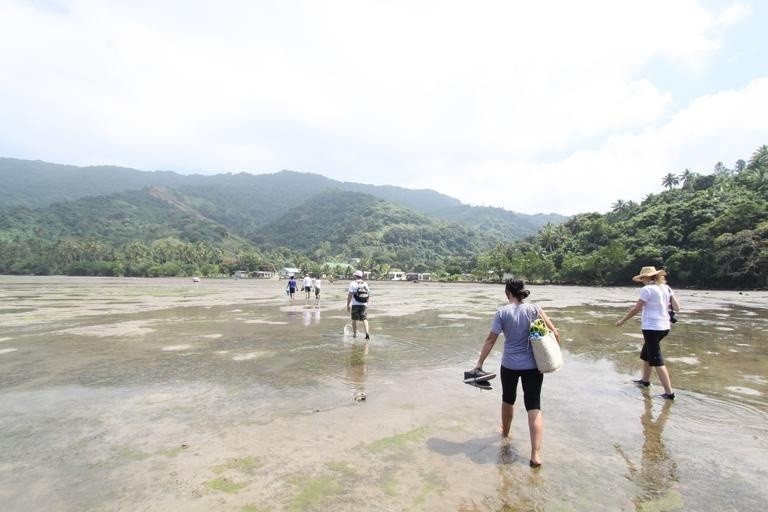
<box><xmin>468</xmin><ymin>380</ymin><xmax>493</xmax><ymax>390</ymax></box>
<box><xmin>658</xmin><ymin>392</ymin><xmax>676</xmax><ymax>400</ymax></box>
<box><xmin>630</xmin><ymin>378</ymin><xmax>651</xmax><ymax>388</ymax></box>
<box><xmin>463</xmin><ymin>366</ymin><xmax>497</xmax><ymax>383</ymax></box>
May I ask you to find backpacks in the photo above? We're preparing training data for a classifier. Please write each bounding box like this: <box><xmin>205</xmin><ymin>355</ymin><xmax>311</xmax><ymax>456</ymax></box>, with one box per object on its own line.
<box><xmin>353</xmin><ymin>281</ymin><xmax>369</xmax><ymax>303</ymax></box>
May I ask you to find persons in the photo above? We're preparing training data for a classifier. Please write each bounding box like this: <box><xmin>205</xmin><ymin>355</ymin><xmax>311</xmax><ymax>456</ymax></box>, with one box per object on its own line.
<box><xmin>426</xmin><ymin>433</ymin><xmax>530</xmax><ymax>466</ymax></box>
<box><xmin>303</xmin><ymin>273</ymin><xmax>313</xmax><ymax>300</ymax></box>
<box><xmin>474</xmin><ymin>277</ymin><xmax>560</xmax><ymax>467</ymax></box>
<box><xmin>612</xmin><ymin>389</ymin><xmax>679</xmax><ymax>512</ymax></box>
<box><xmin>286</xmin><ymin>276</ymin><xmax>298</xmax><ymax>302</ymax></box>
<box><xmin>614</xmin><ymin>266</ymin><xmax>680</xmax><ymax>400</ymax></box>
<box><xmin>314</xmin><ymin>277</ymin><xmax>321</xmax><ymax>300</ymax></box>
<box><xmin>347</xmin><ymin>270</ymin><xmax>370</xmax><ymax>339</ymax></box>
<box><xmin>346</xmin><ymin>340</ymin><xmax>369</xmax><ymax>387</ymax></box>
<box><xmin>288</xmin><ymin>300</ymin><xmax>322</xmax><ymax>325</ymax></box>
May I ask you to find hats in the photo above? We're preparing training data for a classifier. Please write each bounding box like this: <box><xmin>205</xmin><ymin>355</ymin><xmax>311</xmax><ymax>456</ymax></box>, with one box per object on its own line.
<box><xmin>352</xmin><ymin>270</ymin><xmax>363</xmax><ymax>278</ymax></box>
<box><xmin>632</xmin><ymin>265</ymin><xmax>667</xmax><ymax>284</ymax></box>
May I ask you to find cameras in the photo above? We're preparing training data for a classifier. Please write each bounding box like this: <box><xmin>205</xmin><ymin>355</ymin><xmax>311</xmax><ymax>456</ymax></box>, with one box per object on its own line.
<box><xmin>669</xmin><ymin>310</ymin><xmax>677</xmax><ymax>323</ymax></box>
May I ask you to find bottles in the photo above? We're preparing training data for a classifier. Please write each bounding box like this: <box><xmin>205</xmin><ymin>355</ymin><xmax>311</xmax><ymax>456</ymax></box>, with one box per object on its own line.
<box><xmin>530</xmin><ymin>332</ymin><xmax>542</xmax><ymax>339</ymax></box>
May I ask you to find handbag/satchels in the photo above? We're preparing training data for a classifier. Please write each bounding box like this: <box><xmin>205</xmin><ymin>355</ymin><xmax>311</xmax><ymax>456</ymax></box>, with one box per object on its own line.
<box><xmin>526</xmin><ymin>302</ymin><xmax>563</xmax><ymax>375</ymax></box>
